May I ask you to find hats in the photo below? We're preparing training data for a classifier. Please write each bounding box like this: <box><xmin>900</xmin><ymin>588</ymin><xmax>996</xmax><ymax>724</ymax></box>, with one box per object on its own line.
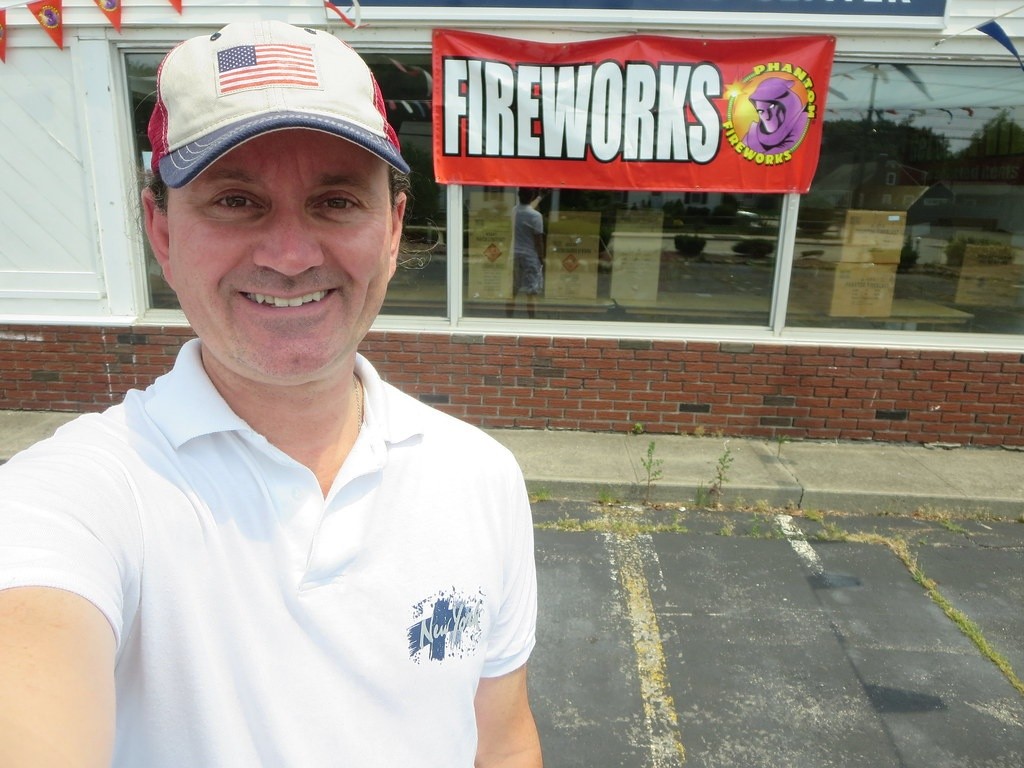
<box><xmin>147</xmin><ymin>19</ymin><xmax>410</xmax><ymax>189</ymax></box>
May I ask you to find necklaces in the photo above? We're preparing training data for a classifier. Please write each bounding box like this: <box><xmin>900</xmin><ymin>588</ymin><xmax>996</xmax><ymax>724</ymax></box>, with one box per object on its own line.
<box><xmin>354</xmin><ymin>376</ymin><xmax>361</xmax><ymax>432</ymax></box>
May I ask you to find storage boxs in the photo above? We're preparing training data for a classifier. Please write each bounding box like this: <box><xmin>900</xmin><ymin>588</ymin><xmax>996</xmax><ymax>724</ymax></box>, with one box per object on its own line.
<box><xmin>814</xmin><ymin>181</ymin><xmax>930</xmax><ymax>263</ymax></box>
<box><xmin>797</xmin><ymin>257</ymin><xmax>899</xmax><ymax>318</ymax></box>
<box><xmin>610</xmin><ymin>207</ymin><xmax>664</xmax><ymax>305</ymax></box>
<box><xmin>468</xmin><ymin>192</ymin><xmax>515</xmax><ymax>298</ymax></box>
<box><xmin>545</xmin><ymin>211</ymin><xmax>601</xmax><ymax>299</ymax></box>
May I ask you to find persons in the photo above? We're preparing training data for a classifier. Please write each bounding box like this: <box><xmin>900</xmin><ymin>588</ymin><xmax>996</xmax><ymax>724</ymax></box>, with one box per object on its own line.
<box><xmin>508</xmin><ymin>187</ymin><xmax>545</xmax><ymax>319</ymax></box>
<box><xmin>0</xmin><ymin>20</ymin><xmax>542</xmax><ymax>768</ymax></box>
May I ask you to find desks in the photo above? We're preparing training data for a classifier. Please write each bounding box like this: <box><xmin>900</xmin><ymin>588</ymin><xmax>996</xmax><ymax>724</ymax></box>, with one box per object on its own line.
<box><xmin>379</xmin><ymin>281</ymin><xmax>615</xmax><ymax>321</ymax></box>
<box><xmin>615</xmin><ymin>289</ymin><xmax>974</xmax><ymax>333</ymax></box>
